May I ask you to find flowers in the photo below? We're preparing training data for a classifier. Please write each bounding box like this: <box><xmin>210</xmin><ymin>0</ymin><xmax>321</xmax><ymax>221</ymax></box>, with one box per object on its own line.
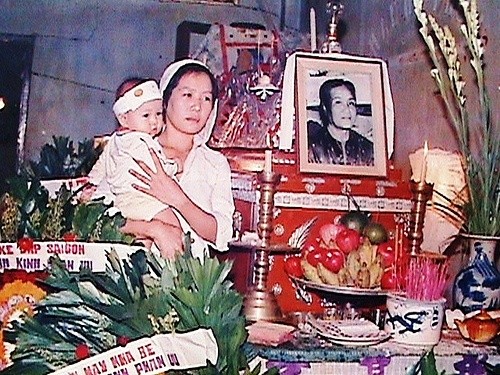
<box><xmin>412</xmin><ymin>0</ymin><xmax>500</xmax><ymax>239</ymax></box>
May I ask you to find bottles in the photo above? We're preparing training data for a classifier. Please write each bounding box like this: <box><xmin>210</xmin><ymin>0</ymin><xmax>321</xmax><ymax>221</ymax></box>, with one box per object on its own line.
<box><xmin>385</xmin><ymin>291</ymin><xmax>446</xmax><ymax>347</ymax></box>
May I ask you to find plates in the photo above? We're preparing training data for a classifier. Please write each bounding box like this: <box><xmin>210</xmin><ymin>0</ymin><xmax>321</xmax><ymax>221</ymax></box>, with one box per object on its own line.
<box><xmin>318</xmin><ymin>327</ymin><xmax>389</xmax><ymax>346</ymax></box>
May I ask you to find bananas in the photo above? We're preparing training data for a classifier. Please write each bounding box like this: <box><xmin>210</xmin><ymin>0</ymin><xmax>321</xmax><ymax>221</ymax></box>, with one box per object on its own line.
<box><xmin>302</xmin><ymin>238</ymin><xmax>385</xmax><ymax>289</ymax></box>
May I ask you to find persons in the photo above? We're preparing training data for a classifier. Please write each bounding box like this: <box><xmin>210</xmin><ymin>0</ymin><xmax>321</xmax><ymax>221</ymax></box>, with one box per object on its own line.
<box><xmin>89</xmin><ymin>56</ymin><xmax>236</xmax><ymax>278</ymax></box>
<box><xmin>309</xmin><ymin>78</ymin><xmax>374</xmax><ymax>166</ymax></box>
<box><xmin>80</xmin><ymin>79</ymin><xmax>183</xmax><ymax>250</ymax></box>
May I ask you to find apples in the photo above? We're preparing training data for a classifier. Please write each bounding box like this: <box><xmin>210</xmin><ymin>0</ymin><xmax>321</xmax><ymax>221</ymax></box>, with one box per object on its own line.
<box><xmin>283</xmin><ymin>225</ymin><xmax>359</xmax><ymax>278</ymax></box>
<box><xmin>377</xmin><ymin>242</ymin><xmax>403</xmax><ymax>289</ymax></box>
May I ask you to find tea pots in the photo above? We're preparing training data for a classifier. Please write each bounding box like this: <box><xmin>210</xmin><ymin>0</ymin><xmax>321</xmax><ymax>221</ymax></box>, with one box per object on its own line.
<box><xmin>454</xmin><ymin>310</ymin><xmax>500</xmax><ymax>342</ymax></box>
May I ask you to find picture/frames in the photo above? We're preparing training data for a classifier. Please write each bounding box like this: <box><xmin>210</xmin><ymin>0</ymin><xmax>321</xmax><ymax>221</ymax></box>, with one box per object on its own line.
<box><xmin>295</xmin><ymin>54</ymin><xmax>389</xmax><ymax>178</ymax></box>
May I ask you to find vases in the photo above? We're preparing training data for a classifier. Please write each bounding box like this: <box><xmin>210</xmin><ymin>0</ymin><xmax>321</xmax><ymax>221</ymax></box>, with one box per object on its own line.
<box><xmin>461</xmin><ymin>231</ymin><xmax>500</xmax><ymax>267</ymax></box>
